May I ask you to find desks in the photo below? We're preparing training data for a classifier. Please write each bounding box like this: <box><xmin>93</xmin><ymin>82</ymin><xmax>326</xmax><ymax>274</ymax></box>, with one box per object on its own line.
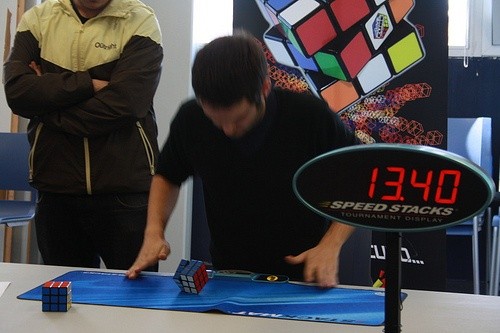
<box><xmin>0</xmin><ymin>262</ymin><xmax>500</xmax><ymax>333</ymax></box>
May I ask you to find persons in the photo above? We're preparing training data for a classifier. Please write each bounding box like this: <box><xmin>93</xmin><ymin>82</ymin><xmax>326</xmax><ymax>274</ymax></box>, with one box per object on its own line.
<box><xmin>125</xmin><ymin>36</ymin><xmax>371</xmax><ymax>287</ymax></box>
<box><xmin>2</xmin><ymin>0</ymin><xmax>162</xmax><ymax>272</ymax></box>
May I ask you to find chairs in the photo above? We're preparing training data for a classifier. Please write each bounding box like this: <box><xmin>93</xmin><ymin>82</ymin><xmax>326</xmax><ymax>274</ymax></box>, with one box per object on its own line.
<box><xmin>472</xmin><ymin>192</ymin><xmax>500</xmax><ymax>297</ymax></box>
<box><xmin>0</xmin><ymin>132</ymin><xmax>40</xmax><ymax>265</ymax></box>
